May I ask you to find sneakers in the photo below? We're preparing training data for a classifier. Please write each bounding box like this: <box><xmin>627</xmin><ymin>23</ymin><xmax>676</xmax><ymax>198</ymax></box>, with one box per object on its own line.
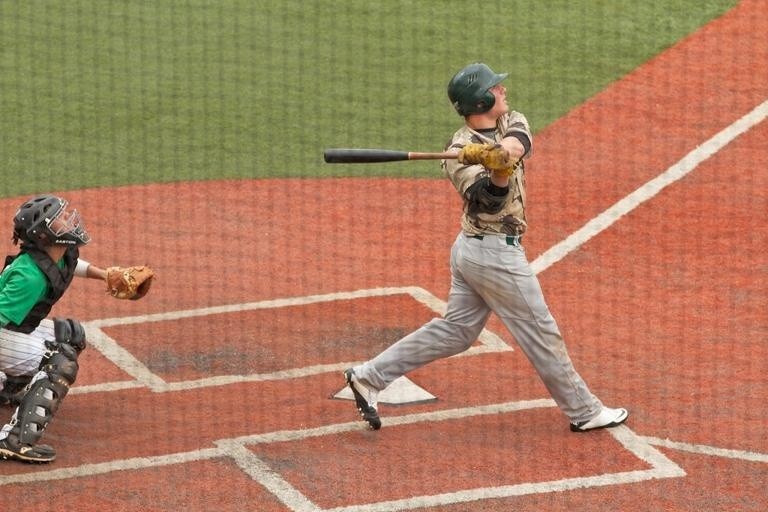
<box><xmin>1</xmin><ymin>387</ymin><xmax>31</xmax><ymax>406</ymax></box>
<box><xmin>570</xmin><ymin>405</ymin><xmax>628</xmax><ymax>431</ymax></box>
<box><xmin>344</xmin><ymin>368</ymin><xmax>382</xmax><ymax>430</ymax></box>
<box><xmin>1</xmin><ymin>432</ymin><xmax>58</xmax><ymax>464</ymax></box>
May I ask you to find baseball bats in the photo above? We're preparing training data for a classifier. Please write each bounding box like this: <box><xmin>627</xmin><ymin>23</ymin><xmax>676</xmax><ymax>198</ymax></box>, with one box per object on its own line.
<box><xmin>322</xmin><ymin>148</ymin><xmax>512</xmax><ymax>163</ymax></box>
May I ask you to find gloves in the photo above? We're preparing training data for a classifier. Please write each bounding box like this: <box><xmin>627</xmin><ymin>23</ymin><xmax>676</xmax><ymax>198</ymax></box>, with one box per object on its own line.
<box><xmin>459</xmin><ymin>143</ymin><xmax>515</xmax><ymax>175</ymax></box>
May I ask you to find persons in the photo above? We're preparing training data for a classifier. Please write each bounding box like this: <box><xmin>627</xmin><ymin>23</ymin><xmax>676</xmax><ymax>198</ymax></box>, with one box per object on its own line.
<box><xmin>345</xmin><ymin>59</ymin><xmax>632</xmax><ymax>435</ymax></box>
<box><xmin>0</xmin><ymin>193</ymin><xmax>154</xmax><ymax>465</ymax></box>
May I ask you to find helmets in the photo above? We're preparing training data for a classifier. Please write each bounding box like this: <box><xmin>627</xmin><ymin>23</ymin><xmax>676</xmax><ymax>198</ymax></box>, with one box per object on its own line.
<box><xmin>14</xmin><ymin>195</ymin><xmax>91</xmax><ymax>250</ymax></box>
<box><xmin>448</xmin><ymin>62</ymin><xmax>511</xmax><ymax>116</ymax></box>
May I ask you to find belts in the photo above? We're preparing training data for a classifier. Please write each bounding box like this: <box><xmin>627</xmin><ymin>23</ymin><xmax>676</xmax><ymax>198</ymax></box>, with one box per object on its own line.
<box><xmin>474</xmin><ymin>234</ymin><xmax>524</xmax><ymax>245</ymax></box>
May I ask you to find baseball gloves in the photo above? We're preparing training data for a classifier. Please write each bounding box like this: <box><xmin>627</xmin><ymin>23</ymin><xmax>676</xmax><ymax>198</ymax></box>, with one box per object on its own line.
<box><xmin>107</xmin><ymin>264</ymin><xmax>152</xmax><ymax>300</ymax></box>
